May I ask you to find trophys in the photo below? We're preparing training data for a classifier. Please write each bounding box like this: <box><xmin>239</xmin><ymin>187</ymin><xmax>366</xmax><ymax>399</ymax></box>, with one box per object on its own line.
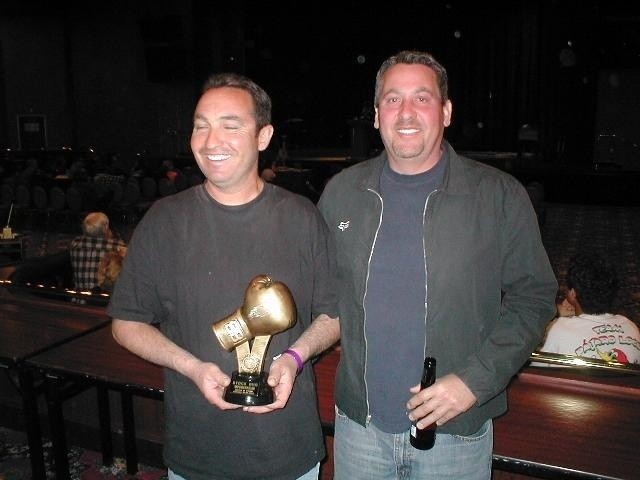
<box><xmin>211</xmin><ymin>275</ymin><xmax>297</xmax><ymax>405</ymax></box>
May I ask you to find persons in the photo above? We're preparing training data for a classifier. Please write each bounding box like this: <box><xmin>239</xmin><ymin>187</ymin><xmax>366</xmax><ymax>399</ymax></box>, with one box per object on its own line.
<box><xmin>105</xmin><ymin>73</ymin><xmax>342</xmax><ymax>480</ymax></box>
<box><xmin>540</xmin><ymin>252</ymin><xmax>638</xmax><ymax>362</ymax></box>
<box><xmin>69</xmin><ymin>213</ymin><xmax>128</xmax><ymax>296</ymax></box>
<box><xmin>316</xmin><ymin>50</ymin><xmax>559</xmax><ymax>480</ymax></box>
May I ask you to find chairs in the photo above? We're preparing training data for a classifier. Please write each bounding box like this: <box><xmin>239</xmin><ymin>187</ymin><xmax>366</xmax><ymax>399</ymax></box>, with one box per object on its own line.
<box><xmin>0</xmin><ymin>152</ymin><xmax>204</xmax><ymax>232</ymax></box>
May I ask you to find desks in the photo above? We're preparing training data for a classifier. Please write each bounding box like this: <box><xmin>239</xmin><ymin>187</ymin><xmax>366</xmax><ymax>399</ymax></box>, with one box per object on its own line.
<box><xmin>0</xmin><ymin>290</ymin><xmax>166</xmax><ymax>480</ymax></box>
<box><xmin>311</xmin><ymin>349</ymin><xmax>639</xmax><ymax>480</ymax></box>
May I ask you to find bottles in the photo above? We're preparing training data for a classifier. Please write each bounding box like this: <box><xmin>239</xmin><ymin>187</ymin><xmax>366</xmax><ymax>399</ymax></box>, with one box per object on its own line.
<box><xmin>410</xmin><ymin>356</ymin><xmax>436</xmax><ymax>451</ymax></box>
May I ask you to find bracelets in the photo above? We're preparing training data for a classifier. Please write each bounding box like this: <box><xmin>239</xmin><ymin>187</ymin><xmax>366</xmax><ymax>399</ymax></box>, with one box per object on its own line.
<box><xmin>282</xmin><ymin>350</ymin><xmax>304</xmax><ymax>372</ymax></box>
